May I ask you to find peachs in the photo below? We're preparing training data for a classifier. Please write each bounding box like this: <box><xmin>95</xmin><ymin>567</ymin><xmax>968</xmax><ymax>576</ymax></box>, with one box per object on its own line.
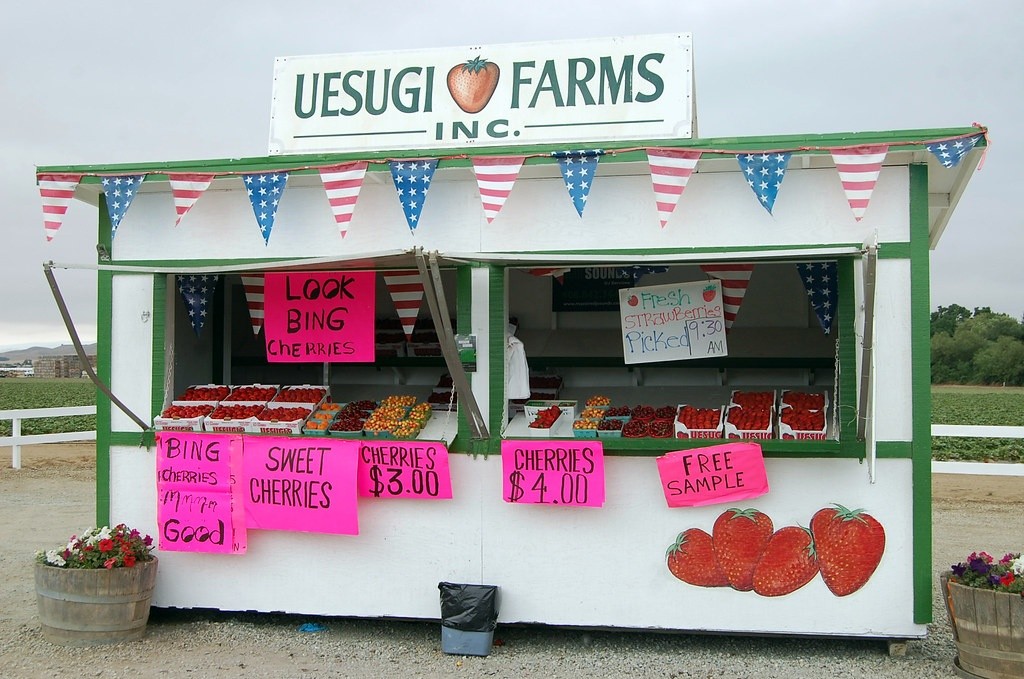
<box><xmin>307</xmin><ymin>402</ymin><xmax>341</xmax><ymax>430</ymax></box>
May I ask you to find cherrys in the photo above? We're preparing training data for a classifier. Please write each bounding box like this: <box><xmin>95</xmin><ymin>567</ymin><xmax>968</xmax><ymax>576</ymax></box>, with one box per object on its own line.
<box><xmin>572</xmin><ymin>395</ymin><xmax>677</xmax><ymax>437</ymax></box>
<box><xmin>329</xmin><ymin>393</ymin><xmax>431</xmax><ymax>438</ymax></box>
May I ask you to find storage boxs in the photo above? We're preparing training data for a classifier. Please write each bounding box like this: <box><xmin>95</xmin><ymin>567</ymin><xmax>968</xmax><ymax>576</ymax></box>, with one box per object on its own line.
<box><xmin>153</xmin><ymin>400</ymin><xmax>217</xmax><ymax>432</ymax></box>
<box><xmin>302</xmin><ymin>400</ymin><xmax>433</xmax><ymax>440</ymax></box>
<box><xmin>780</xmin><ymin>390</ymin><xmax>830</xmax><ymax>412</ymax></box>
<box><xmin>222</xmin><ymin>384</ymin><xmax>280</xmax><ymax>402</ymax></box>
<box><xmin>269</xmin><ymin>385</ymin><xmax>331</xmax><ymax>408</ymax></box>
<box><xmin>528</xmin><ymin>411</ymin><xmax>565</xmax><ymax>437</ymax></box>
<box><xmin>188</xmin><ymin>383</ymin><xmax>234</xmax><ymax>406</ymax></box>
<box><xmin>425</xmin><ymin>388</ymin><xmax>457</xmax><ymax>411</ymax></box>
<box><xmin>778</xmin><ymin>405</ymin><xmax>828</xmax><ymax>440</ymax></box>
<box><xmin>509</xmin><ymin>373</ymin><xmax>565</xmax><ymax>418</ymax></box>
<box><xmin>729</xmin><ymin>389</ymin><xmax>778</xmax><ymax>417</ymax></box>
<box><xmin>203</xmin><ymin>401</ymin><xmax>268</xmax><ymax>432</ymax></box>
<box><xmin>571</xmin><ymin>399</ymin><xmax>726</xmax><ymax>440</ymax></box>
<box><xmin>375</xmin><ymin>322</ymin><xmax>518</xmax><ymax>358</ymax></box>
<box><xmin>524</xmin><ymin>399</ymin><xmax>579</xmax><ymax>420</ymax></box>
<box><xmin>724</xmin><ymin>406</ymin><xmax>773</xmax><ymax>440</ymax></box>
<box><xmin>250</xmin><ymin>402</ymin><xmax>316</xmax><ymax>434</ymax></box>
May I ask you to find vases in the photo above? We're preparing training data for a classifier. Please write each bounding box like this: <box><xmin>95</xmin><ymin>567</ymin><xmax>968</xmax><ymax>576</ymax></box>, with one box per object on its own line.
<box><xmin>939</xmin><ymin>569</ymin><xmax>1024</xmax><ymax>679</ymax></box>
<box><xmin>34</xmin><ymin>554</ymin><xmax>160</xmax><ymax>648</ymax></box>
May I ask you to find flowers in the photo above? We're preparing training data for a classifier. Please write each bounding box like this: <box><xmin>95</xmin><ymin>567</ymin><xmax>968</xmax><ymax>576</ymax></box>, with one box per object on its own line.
<box><xmin>34</xmin><ymin>523</ymin><xmax>157</xmax><ymax>570</ymax></box>
<box><xmin>951</xmin><ymin>551</ymin><xmax>1024</xmax><ymax>598</ymax></box>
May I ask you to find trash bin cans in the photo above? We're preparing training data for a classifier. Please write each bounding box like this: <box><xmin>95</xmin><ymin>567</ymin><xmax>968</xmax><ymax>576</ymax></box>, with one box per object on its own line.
<box><xmin>438</xmin><ymin>581</ymin><xmax>502</xmax><ymax>657</ymax></box>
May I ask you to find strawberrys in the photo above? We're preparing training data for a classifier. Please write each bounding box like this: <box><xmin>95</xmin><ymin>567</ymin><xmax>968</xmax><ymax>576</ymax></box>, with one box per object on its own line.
<box><xmin>528</xmin><ymin>405</ymin><xmax>562</xmax><ymax>428</ymax></box>
